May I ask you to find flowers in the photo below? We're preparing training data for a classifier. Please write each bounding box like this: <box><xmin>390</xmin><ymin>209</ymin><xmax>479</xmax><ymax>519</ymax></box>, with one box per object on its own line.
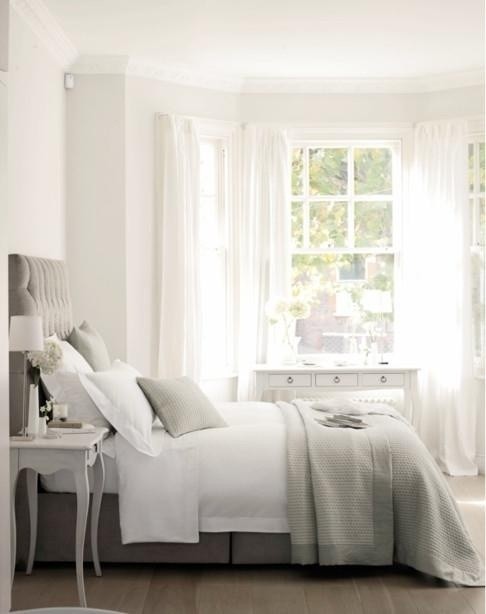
<box><xmin>24</xmin><ymin>339</ymin><xmax>62</xmax><ymax>389</ymax></box>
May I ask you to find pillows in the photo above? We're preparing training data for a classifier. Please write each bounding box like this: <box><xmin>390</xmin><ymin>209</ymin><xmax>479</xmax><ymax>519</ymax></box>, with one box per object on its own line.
<box><xmin>76</xmin><ymin>359</ymin><xmax>158</xmax><ymax>458</ymax></box>
<box><xmin>136</xmin><ymin>377</ymin><xmax>230</xmax><ymax>438</ymax></box>
<box><xmin>66</xmin><ymin>320</ymin><xmax>111</xmax><ymax>372</ymax></box>
<box><xmin>8</xmin><ymin>254</ymin><xmax>486</xmax><ymax>588</ymax></box>
<box><xmin>28</xmin><ymin>332</ymin><xmax>111</xmax><ymax>426</ymax></box>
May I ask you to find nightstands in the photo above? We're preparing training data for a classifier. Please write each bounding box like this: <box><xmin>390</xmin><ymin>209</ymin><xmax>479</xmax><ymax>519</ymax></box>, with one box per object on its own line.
<box><xmin>9</xmin><ymin>426</ymin><xmax>110</xmax><ymax>609</ymax></box>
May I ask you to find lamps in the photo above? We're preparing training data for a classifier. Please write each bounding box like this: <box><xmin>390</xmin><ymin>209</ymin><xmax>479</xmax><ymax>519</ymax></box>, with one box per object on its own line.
<box><xmin>8</xmin><ymin>315</ymin><xmax>44</xmax><ymax>437</ymax></box>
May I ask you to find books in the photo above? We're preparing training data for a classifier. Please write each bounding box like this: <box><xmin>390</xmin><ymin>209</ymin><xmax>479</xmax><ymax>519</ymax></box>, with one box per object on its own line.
<box><xmin>315</xmin><ymin>413</ymin><xmax>371</xmax><ymax>430</ymax></box>
<box><xmin>48</xmin><ymin>420</ymin><xmax>94</xmax><ymax>433</ymax></box>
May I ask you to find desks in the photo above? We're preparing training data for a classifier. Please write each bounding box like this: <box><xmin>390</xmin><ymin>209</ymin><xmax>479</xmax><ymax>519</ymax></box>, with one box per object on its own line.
<box><xmin>252</xmin><ymin>354</ymin><xmax>421</xmax><ymax>434</ymax></box>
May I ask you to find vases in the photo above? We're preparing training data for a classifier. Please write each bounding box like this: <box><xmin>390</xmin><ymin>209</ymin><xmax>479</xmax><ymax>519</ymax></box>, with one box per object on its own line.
<box><xmin>27</xmin><ymin>383</ymin><xmax>40</xmax><ymax>434</ymax></box>
